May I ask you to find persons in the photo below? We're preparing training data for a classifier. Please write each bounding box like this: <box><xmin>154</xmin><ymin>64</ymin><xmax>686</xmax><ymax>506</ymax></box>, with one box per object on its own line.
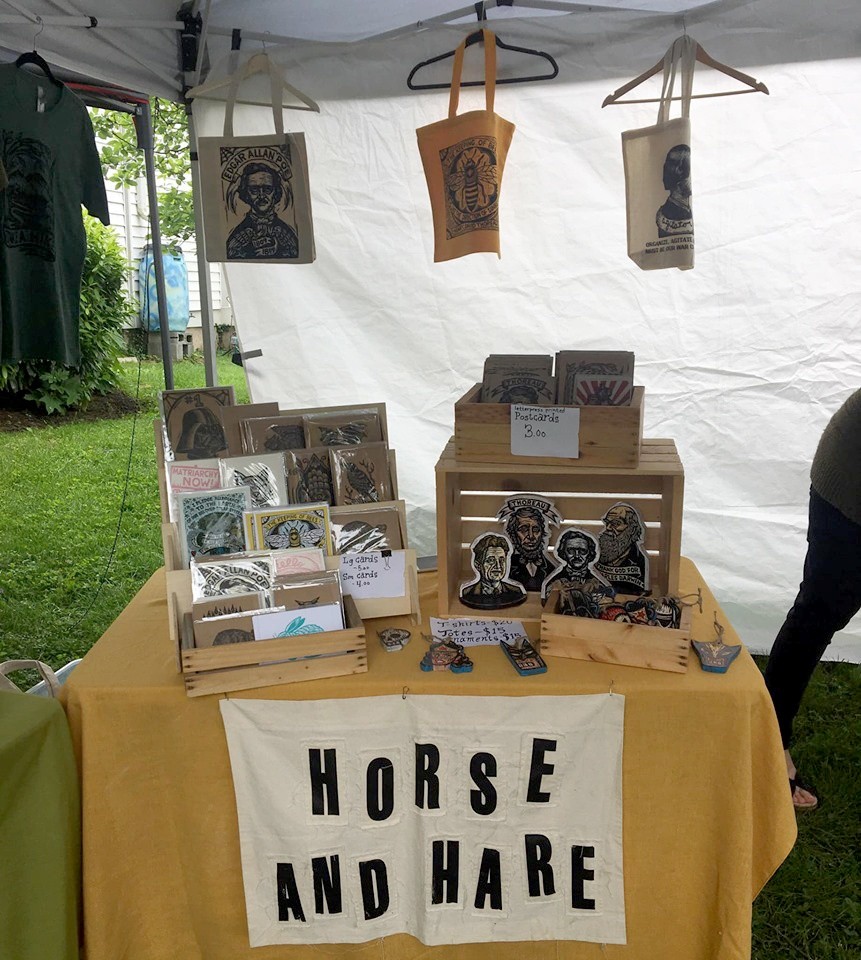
<box><xmin>764</xmin><ymin>388</ymin><xmax>861</xmax><ymax>808</ymax></box>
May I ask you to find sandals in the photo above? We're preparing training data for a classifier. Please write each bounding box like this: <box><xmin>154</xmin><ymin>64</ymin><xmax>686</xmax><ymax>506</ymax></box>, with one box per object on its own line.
<box><xmin>789</xmin><ymin>771</ymin><xmax>818</xmax><ymax>811</ymax></box>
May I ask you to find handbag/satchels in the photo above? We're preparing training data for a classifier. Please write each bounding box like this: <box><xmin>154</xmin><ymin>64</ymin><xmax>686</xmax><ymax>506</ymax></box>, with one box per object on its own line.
<box><xmin>0</xmin><ymin>658</ymin><xmax>83</xmax><ymax>699</ymax></box>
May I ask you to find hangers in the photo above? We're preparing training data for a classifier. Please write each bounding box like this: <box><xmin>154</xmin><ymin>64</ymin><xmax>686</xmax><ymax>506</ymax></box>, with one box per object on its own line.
<box><xmin>602</xmin><ymin>14</ymin><xmax>769</xmax><ymax>107</ymax></box>
<box><xmin>16</xmin><ymin>17</ymin><xmax>63</xmax><ymax>88</ymax></box>
<box><xmin>407</xmin><ymin>2</ymin><xmax>558</xmax><ymax>90</ymax></box>
<box><xmin>185</xmin><ymin>30</ymin><xmax>320</xmax><ymax>112</ymax></box>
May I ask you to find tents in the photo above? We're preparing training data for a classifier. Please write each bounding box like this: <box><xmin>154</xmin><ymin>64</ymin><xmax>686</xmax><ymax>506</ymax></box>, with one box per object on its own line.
<box><xmin>0</xmin><ymin>0</ymin><xmax>861</xmax><ymax>390</ymax></box>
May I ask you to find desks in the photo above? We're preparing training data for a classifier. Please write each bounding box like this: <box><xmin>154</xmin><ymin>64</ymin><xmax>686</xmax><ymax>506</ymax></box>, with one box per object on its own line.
<box><xmin>1</xmin><ymin>690</ymin><xmax>82</xmax><ymax>960</ymax></box>
<box><xmin>62</xmin><ymin>565</ymin><xmax>797</xmax><ymax>960</ymax></box>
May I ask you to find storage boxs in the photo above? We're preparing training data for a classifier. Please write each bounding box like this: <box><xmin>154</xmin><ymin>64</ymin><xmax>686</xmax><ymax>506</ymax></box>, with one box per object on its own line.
<box><xmin>540</xmin><ymin>590</ymin><xmax>694</xmax><ymax>673</ymax></box>
<box><xmin>181</xmin><ymin>594</ymin><xmax>369</xmax><ymax>698</ymax></box>
<box><xmin>436</xmin><ymin>436</ymin><xmax>685</xmax><ymax>623</ymax></box>
<box><xmin>455</xmin><ymin>383</ymin><xmax>645</xmax><ymax>469</ymax></box>
